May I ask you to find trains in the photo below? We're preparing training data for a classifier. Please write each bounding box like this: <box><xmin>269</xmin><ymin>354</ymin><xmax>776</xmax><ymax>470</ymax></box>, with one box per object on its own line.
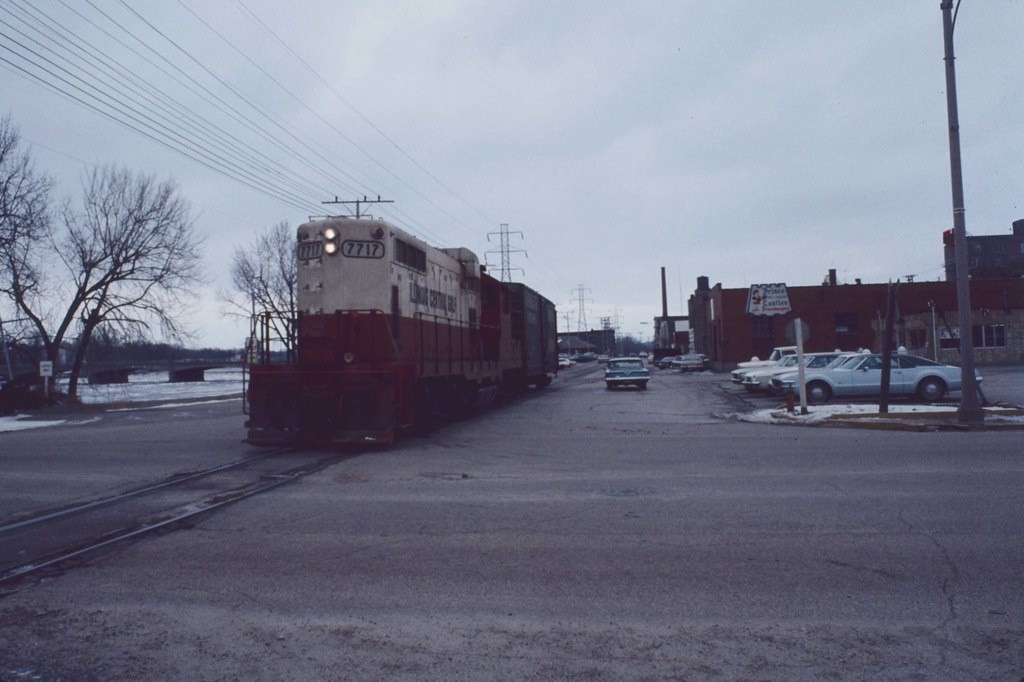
<box><xmin>241</xmin><ymin>213</ymin><xmax>560</xmax><ymax>448</ymax></box>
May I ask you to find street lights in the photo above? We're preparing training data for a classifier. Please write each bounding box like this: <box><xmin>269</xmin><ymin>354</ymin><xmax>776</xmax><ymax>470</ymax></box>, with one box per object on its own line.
<box><xmin>928</xmin><ymin>299</ymin><xmax>938</xmax><ymax>362</ymax></box>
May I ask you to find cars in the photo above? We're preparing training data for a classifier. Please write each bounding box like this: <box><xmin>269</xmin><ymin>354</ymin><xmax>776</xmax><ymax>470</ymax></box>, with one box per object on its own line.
<box><xmin>653</xmin><ymin>357</ymin><xmax>674</xmax><ymax>370</ymax></box>
<box><xmin>730</xmin><ymin>352</ymin><xmax>859</xmax><ymax>396</ymax></box>
<box><xmin>737</xmin><ymin>346</ymin><xmax>798</xmax><ymax>369</ymax></box>
<box><xmin>681</xmin><ymin>356</ymin><xmax>704</xmax><ymax>372</ymax></box>
<box><xmin>585</xmin><ymin>352</ymin><xmax>598</xmax><ymax>359</ymax></box>
<box><xmin>668</xmin><ymin>356</ymin><xmax>682</xmax><ymax>368</ymax></box>
<box><xmin>768</xmin><ymin>353</ymin><xmax>983</xmax><ymax>404</ymax></box>
<box><xmin>598</xmin><ymin>355</ymin><xmax>609</xmax><ymax>363</ymax></box>
<box><xmin>558</xmin><ymin>357</ymin><xmax>571</xmax><ymax>367</ymax></box>
<box><xmin>640</xmin><ymin>351</ymin><xmax>647</xmax><ymax>358</ymax></box>
<box><xmin>648</xmin><ymin>355</ymin><xmax>654</xmax><ymax>364</ymax></box>
<box><xmin>570</xmin><ymin>354</ymin><xmax>593</xmax><ymax>362</ymax></box>
<box><xmin>559</xmin><ymin>353</ymin><xmax>568</xmax><ymax>358</ymax></box>
<box><xmin>604</xmin><ymin>357</ymin><xmax>650</xmax><ymax>389</ymax></box>
